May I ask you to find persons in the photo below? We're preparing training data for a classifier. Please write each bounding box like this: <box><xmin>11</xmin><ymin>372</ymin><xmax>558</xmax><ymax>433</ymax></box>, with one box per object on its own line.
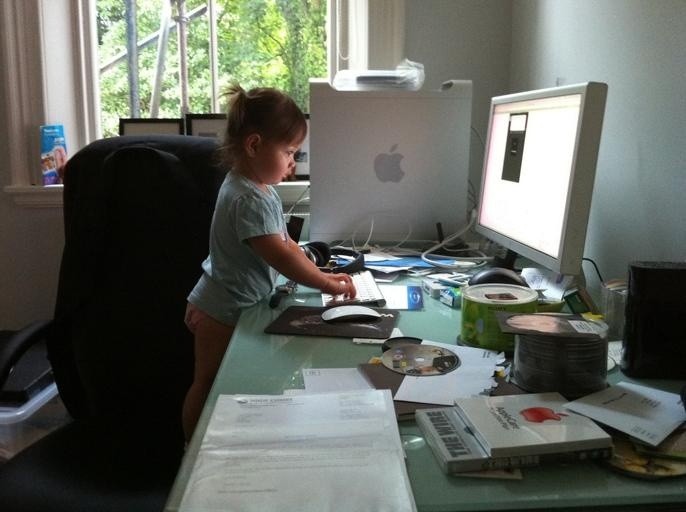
<box><xmin>181</xmin><ymin>87</ymin><xmax>357</xmax><ymax>453</ymax></box>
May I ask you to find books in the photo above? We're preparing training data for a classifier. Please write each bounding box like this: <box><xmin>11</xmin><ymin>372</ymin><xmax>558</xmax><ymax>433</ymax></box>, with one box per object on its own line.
<box><xmin>414</xmin><ymin>405</ymin><xmax>614</xmax><ymax>475</ymax></box>
<box><xmin>452</xmin><ymin>391</ymin><xmax>612</xmax><ymax>457</ymax></box>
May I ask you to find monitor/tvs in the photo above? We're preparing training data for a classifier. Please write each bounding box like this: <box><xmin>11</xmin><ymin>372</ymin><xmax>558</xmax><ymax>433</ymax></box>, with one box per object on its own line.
<box><xmin>474</xmin><ymin>81</ymin><xmax>608</xmax><ymax>277</ymax></box>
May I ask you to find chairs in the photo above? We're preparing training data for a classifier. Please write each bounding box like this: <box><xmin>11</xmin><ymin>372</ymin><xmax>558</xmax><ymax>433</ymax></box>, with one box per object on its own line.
<box><xmin>1</xmin><ymin>136</ymin><xmax>236</xmax><ymax>511</ymax></box>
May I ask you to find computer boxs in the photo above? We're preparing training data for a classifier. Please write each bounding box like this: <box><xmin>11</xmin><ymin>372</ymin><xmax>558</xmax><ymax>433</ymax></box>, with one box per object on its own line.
<box><xmin>310</xmin><ymin>79</ymin><xmax>475</xmax><ymax>243</ymax></box>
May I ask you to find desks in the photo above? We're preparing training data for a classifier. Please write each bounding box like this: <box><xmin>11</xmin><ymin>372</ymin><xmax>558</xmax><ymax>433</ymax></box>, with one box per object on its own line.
<box><xmin>163</xmin><ymin>243</ymin><xmax>685</xmax><ymax>511</ymax></box>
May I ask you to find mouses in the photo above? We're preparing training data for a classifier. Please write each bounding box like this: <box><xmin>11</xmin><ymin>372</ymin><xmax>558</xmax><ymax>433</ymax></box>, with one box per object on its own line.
<box><xmin>321</xmin><ymin>305</ymin><xmax>383</xmax><ymax>324</ymax></box>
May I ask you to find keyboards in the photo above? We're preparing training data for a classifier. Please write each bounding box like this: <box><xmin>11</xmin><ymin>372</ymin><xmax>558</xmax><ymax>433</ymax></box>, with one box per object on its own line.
<box><xmin>321</xmin><ymin>269</ymin><xmax>385</xmax><ymax>307</ymax></box>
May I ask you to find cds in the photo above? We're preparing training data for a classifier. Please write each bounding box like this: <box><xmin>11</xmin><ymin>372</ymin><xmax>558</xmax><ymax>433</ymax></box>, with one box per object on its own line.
<box><xmin>381</xmin><ymin>344</ymin><xmax>461</xmax><ymax>377</ymax></box>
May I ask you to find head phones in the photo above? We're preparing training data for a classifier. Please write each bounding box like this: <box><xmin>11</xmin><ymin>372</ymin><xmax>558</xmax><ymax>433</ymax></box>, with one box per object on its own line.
<box><xmin>297</xmin><ymin>241</ymin><xmax>364</xmax><ymax>274</ymax></box>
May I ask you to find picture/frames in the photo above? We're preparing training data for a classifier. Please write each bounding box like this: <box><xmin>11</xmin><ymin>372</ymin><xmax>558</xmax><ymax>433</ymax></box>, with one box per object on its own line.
<box><xmin>185</xmin><ymin>113</ymin><xmax>228</xmax><ymax>137</ymax></box>
<box><xmin>118</xmin><ymin>118</ymin><xmax>184</xmax><ymax>136</ymax></box>
<box><xmin>290</xmin><ymin>115</ymin><xmax>310</xmax><ymax>180</ymax></box>
